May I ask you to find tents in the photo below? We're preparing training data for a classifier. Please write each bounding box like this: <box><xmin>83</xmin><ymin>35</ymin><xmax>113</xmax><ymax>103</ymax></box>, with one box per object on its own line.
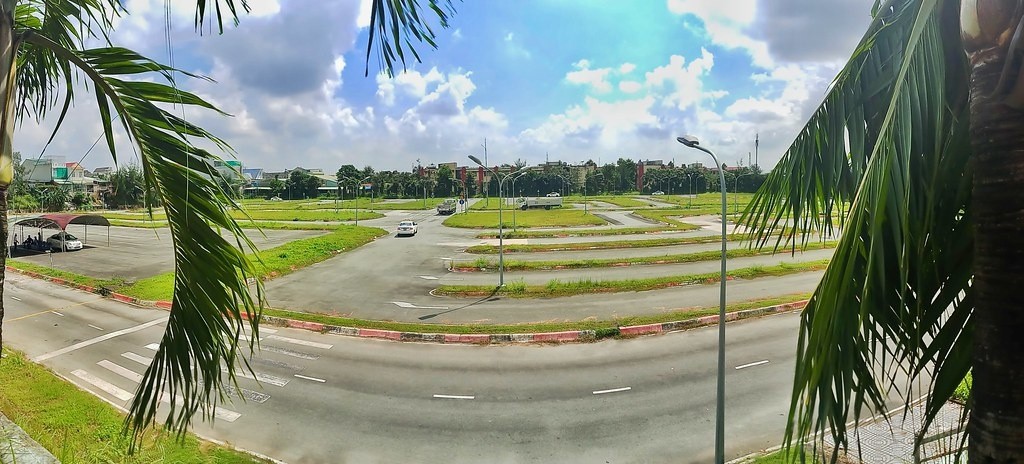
<box><xmin>7</xmin><ymin>213</ymin><xmax>111</xmax><ymax>259</ymax></box>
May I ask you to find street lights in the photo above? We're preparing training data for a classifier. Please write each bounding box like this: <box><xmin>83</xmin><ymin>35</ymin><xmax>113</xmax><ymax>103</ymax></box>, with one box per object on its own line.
<box><xmin>677</xmin><ymin>135</ymin><xmax>726</xmax><ymax>464</ymax></box>
<box><xmin>283</xmin><ymin>182</ymin><xmax>296</xmax><ymax>202</ymax></box>
<box><xmin>448</xmin><ymin>178</ymin><xmax>466</xmax><ymax>213</ymax></box>
<box><xmin>344</xmin><ymin>176</ymin><xmax>370</xmax><ymax>226</ymax></box>
<box><xmin>41</xmin><ymin>188</ymin><xmax>48</xmax><ymax>214</ymax></box>
<box><xmin>103</xmin><ymin>191</ymin><xmax>111</xmax><ymax>212</ymax></box>
<box><xmin>135</xmin><ymin>186</ymin><xmax>154</xmax><ymax>223</ymax></box>
<box><xmin>722</xmin><ymin>164</ymin><xmax>754</xmax><ymax>220</ymax></box>
<box><xmin>468</xmin><ymin>155</ymin><xmax>530</xmax><ymax>284</ymax></box>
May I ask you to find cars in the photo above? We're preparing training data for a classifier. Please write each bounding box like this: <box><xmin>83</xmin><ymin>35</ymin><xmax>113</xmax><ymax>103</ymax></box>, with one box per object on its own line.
<box><xmin>271</xmin><ymin>196</ymin><xmax>283</xmax><ymax>201</ymax></box>
<box><xmin>652</xmin><ymin>190</ymin><xmax>664</xmax><ymax>195</ymax></box>
<box><xmin>397</xmin><ymin>221</ymin><xmax>418</xmax><ymax>236</ymax></box>
<box><xmin>48</xmin><ymin>232</ymin><xmax>83</xmax><ymax>251</ymax></box>
<box><xmin>546</xmin><ymin>192</ymin><xmax>560</xmax><ymax>197</ymax></box>
<box><xmin>585</xmin><ymin>173</ymin><xmax>604</xmax><ymax>214</ymax></box>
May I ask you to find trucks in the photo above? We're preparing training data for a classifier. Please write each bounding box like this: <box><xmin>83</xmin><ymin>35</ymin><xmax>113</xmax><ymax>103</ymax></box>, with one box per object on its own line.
<box><xmin>436</xmin><ymin>198</ymin><xmax>456</xmax><ymax>215</ymax></box>
<box><xmin>516</xmin><ymin>197</ymin><xmax>562</xmax><ymax>210</ymax></box>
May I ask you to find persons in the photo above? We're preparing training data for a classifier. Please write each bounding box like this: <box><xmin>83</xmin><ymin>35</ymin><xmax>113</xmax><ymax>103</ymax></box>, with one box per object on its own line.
<box><xmin>31</xmin><ymin>236</ymin><xmax>37</xmax><ymax>245</ymax></box>
<box><xmin>13</xmin><ymin>233</ymin><xmax>19</xmax><ymax>250</ymax></box>
<box><xmin>37</xmin><ymin>232</ymin><xmax>43</xmax><ymax>247</ymax></box>
<box><xmin>23</xmin><ymin>235</ymin><xmax>32</xmax><ymax>248</ymax></box>
